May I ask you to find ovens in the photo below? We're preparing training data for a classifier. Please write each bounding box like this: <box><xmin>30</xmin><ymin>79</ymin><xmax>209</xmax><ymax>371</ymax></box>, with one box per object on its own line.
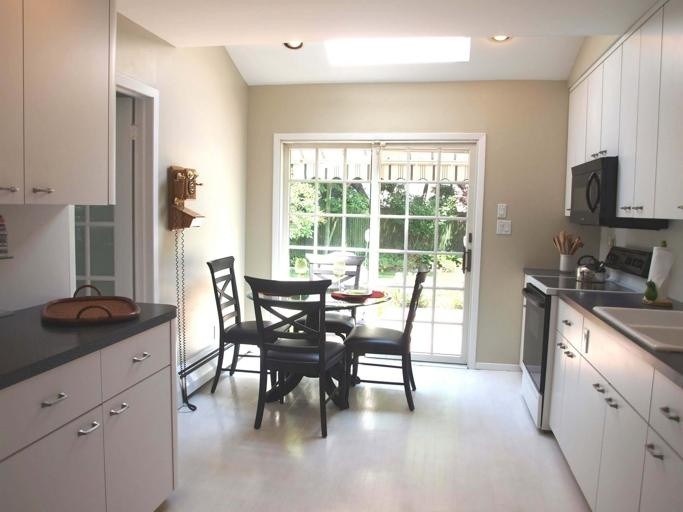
<box><xmin>518</xmin><ymin>283</ymin><xmax>550</xmax><ymax>429</ymax></box>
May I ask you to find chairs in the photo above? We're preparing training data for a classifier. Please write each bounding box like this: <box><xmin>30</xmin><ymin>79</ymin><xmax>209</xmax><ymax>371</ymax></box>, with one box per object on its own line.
<box><xmin>242</xmin><ymin>273</ymin><xmax>343</xmax><ymax>439</ymax></box>
<box><xmin>205</xmin><ymin>255</ymin><xmax>279</xmax><ymax>402</ymax></box>
<box><xmin>339</xmin><ymin>265</ymin><xmax>425</xmax><ymax>413</ymax></box>
<box><xmin>304</xmin><ymin>251</ymin><xmax>364</xmax><ymax>383</ymax></box>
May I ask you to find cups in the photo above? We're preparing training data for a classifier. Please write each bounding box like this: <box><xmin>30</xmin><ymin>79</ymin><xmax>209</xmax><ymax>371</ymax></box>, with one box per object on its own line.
<box><xmin>559</xmin><ymin>253</ymin><xmax>576</xmax><ymax>274</ymax></box>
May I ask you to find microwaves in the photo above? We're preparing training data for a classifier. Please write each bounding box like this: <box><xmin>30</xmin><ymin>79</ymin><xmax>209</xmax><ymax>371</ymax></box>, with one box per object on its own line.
<box><xmin>570</xmin><ymin>156</ymin><xmax>670</xmax><ymax>230</ymax></box>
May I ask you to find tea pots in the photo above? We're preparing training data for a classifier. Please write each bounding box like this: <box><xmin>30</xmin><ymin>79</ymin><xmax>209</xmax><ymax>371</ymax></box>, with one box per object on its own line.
<box><xmin>576</xmin><ymin>255</ymin><xmax>608</xmax><ymax>283</ymax></box>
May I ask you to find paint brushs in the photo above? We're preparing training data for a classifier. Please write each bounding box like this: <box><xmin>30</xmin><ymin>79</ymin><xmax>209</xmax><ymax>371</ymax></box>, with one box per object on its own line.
<box><xmin>553</xmin><ymin>230</ymin><xmax>583</xmax><ymax>255</ymax></box>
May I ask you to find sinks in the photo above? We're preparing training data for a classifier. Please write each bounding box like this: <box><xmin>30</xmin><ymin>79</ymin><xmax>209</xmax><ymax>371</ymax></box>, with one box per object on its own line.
<box><xmin>593</xmin><ymin>304</ymin><xmax>682</xmax><ymax>327</ymax></box>
<box><xmin>623</xmin><ymin>327</ymin><xmax>683</xmax><ymax>354</ymax></box>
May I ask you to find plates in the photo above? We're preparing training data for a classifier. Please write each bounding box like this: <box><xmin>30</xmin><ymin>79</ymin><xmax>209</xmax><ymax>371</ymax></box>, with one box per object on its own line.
<box><xmin>337</xmin><ymin>289</ymin><xmax>372</xmax><ymax>298</ymax></box>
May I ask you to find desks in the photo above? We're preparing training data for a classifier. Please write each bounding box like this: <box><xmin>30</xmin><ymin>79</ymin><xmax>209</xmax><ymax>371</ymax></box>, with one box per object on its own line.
<box><xmin>242</xmin><ymin>281</ymin><xmax>391</xmax><ymax>411</ymax></box>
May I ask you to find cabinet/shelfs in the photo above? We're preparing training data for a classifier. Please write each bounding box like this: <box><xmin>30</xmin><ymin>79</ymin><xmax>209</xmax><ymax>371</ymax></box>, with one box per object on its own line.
<box><xmin>662</xmin><ymin>0</ymin><xmax>683</xmax><ymax>219</ymax></box>
<box><xmin>614</xmin><ymin>5</ymin><xmax>662</xmax><ymax>222</ymax></box>
<box><xmin>582</xmin><ymin>42</ymin><xmax>620</xmax><ymax>160</ymax></box>
<box><xmin>559</xmin><ymin>75</ymin><xmax>582</xmax><ymax>215</ymax></box>
<box><xmin>566</xmin><ymin>317</ymin><xmax>654</xmax><ymax>512</ymax></box>
<box><xmin>640</xmin><ymin>364</ymin><xmax>682</xmax><ymax>512</ymax></box>
<box><xmin>0</xmin><ymin>0</ymin><xmax>121</xmax><ymax>214</ymax></box>
<box><xmin>0</xmin><ymin>309</ymin><xmax>179</xmax><ymax>512</ymax></box>
<box><xmin>546</xmin><ymin>290</ymin><xmax>581</xmax><ymax>488</ymax></box>
